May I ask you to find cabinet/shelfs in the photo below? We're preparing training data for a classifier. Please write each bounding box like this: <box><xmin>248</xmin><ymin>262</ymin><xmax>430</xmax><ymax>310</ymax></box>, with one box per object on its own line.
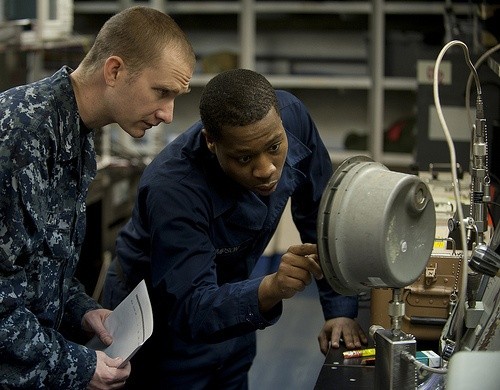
<box><xmin>72</xmin><ymin>0</ymin><xmax>475</xmax><ymax>254</ymax></box>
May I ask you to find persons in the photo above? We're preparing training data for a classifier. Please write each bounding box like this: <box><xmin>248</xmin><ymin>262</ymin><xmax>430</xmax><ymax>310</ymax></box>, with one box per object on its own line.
<box><xmin>0</xmin><ymin>7</ymin><xmax>196</xmax><ymax>390</ymax></box>
<box><xmin>98</xmin><ymin>68</ymin><xmax>369</xmax><ymax>390</ymax></box>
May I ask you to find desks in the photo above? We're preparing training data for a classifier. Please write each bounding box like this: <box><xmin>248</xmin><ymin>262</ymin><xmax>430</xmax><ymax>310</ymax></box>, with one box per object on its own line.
<box><xmin>315</xmin><ymin>306</ymin><xmax>500</xmax><ymax>390</ymax></box>
